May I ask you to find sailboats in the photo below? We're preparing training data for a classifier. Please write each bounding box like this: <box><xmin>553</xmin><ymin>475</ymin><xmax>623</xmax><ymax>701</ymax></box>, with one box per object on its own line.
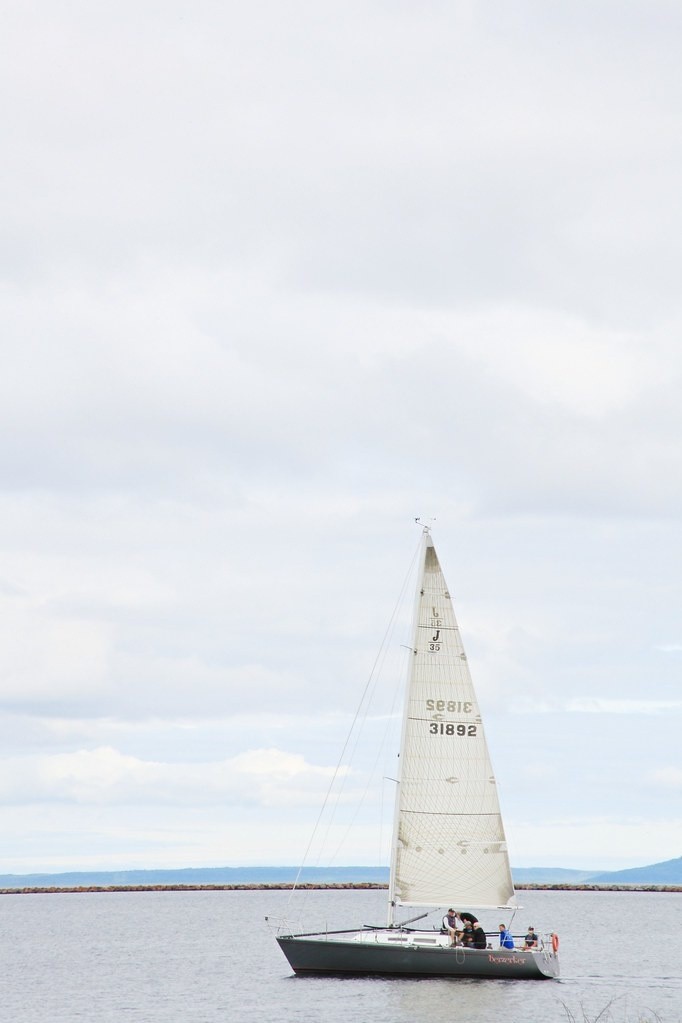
<box><xmin>270</xmin><ymin>519</ymin><xmax>567</xmax><ymax>981</ymax></box>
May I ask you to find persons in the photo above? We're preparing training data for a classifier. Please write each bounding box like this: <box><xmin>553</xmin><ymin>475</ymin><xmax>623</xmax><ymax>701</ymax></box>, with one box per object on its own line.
<box><xmin>442</xmin><ymin>908</ymin><xmax>486</xmax><ymax>949</ymax></box>
<box><xmin>522</xmin><ymin>926</ymin><xmax>538</xmax><ymax>951</ymax></box>
<box><xmin>499</xmin><ymin>924</ymin><xmax>514</xmax><ymax>949</ymax></box>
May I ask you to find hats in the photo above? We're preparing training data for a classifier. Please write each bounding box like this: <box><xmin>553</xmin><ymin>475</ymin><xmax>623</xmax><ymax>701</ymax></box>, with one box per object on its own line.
<box><xmin>448</xmin><ymin>908</ymin><xmax>456</xmax><ymax>912</ymax></box>
<box><xmin>528</xmin><ymin>926</ymin><xmax>534</xmax><ymax>931</ymax></box>
<box><xmin>463</xmin><ymin>921</ymin><xmax>472</xmax><ymax>927</ymax></box>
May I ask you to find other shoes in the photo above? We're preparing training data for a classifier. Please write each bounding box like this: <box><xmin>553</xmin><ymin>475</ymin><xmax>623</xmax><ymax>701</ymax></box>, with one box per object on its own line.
<box><xmin>455</xmin><ymin>942</ymin><xmax>462</xmax><ymax>945</ymax></box>
<box><xmin>451</xmin><ymin>943</ymin><xmax>456</xmax><ymax>947</ymax></box>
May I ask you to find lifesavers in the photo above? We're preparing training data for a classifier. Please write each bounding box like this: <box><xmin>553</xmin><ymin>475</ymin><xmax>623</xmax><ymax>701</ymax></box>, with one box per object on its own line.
<box><xmin>552</xmin><ymin>933</ymin><xmax>559</xmax><ymax>952</ymax></box>
